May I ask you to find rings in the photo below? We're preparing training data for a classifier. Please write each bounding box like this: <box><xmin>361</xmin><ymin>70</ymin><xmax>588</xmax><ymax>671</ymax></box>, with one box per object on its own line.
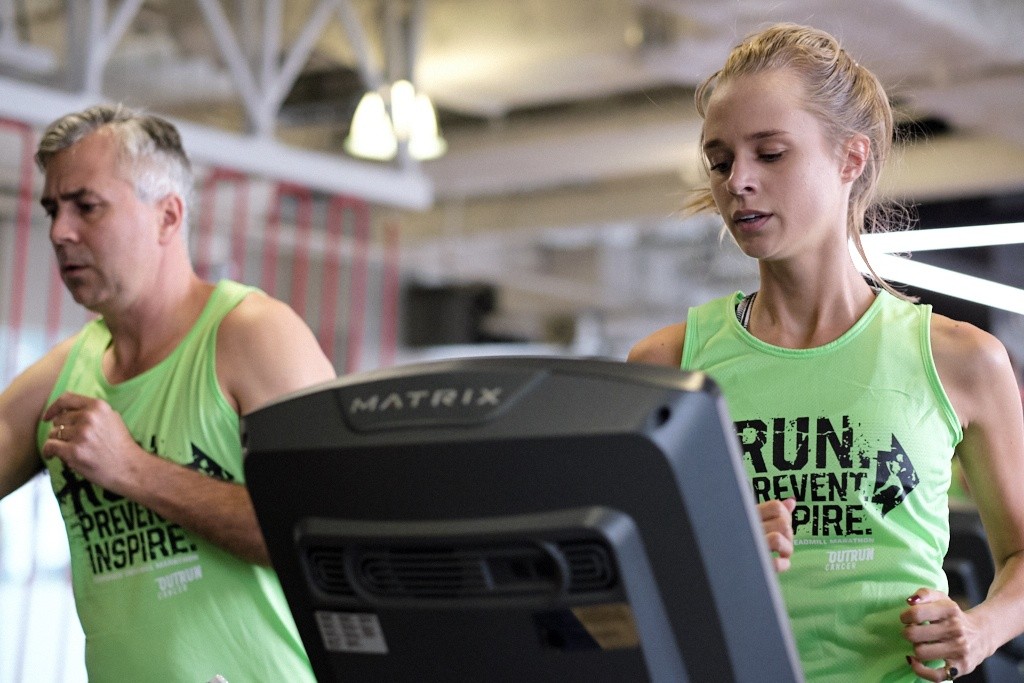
<box><xmin>945</xmin><ymin>666</ymin><xmax>957</xmax><ymax>680</ymax></box>
<box><xmin>57</xmin><ymin>425</ymin><xmax>65</xmax><ymax>442</ymax></box>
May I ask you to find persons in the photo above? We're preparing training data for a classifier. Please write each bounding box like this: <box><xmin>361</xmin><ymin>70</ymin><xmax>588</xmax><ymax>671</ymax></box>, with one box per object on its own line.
<box><xmin>0</xmin><ymin>98</ymin><xmax>337</xmax><ymax>683</ymax></box>
<box><xmin>625</xmin><ymin>17</ymin><xmax>1023</xmax><ymax>683</ymax></box>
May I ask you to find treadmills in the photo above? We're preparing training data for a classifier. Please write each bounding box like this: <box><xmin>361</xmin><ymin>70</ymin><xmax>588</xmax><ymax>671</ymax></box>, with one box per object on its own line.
<box><xmin>237</xmin><ymin>354</ymin><xmax>807</xmax><ymax>683</ymax></box>
